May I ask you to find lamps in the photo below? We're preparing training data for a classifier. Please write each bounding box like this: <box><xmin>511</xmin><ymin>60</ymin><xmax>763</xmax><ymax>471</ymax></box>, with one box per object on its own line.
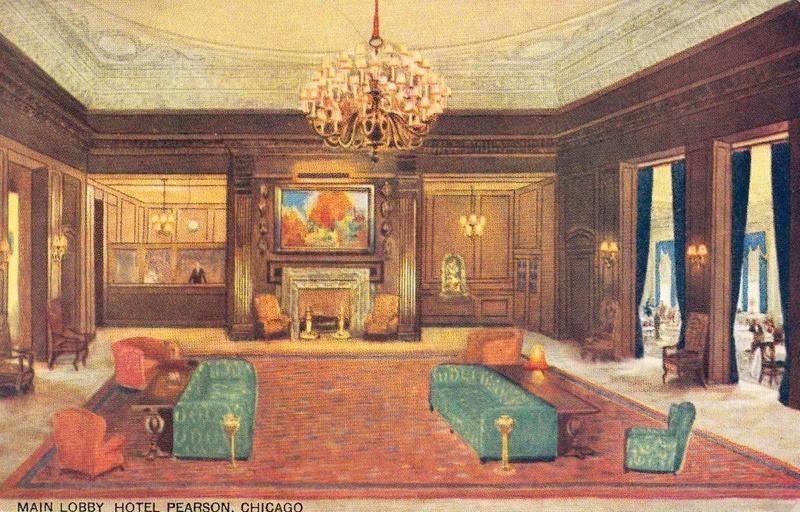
<box><xmin>300</xmin><ymin>1</ymin><xmax>453</xmax><ymax>163</ymax></box>
<box><xmin>458</xmin><ymin>187</ymin><xmax>486</xmax><ymax>239</ymax></box>
<box><xmin>151</xmin><ymin>178</ymin><xmax>175</xmax><ymax>236</ymax></box>
<box><xmin>687</xmin><ymin>245</ymin><xmax>709</xmax><ymax>274</ymax></box>
<box><xmin>597</xmin><ymin>241</ymin><xmax>618</xmax><ymax>271</ymax></box>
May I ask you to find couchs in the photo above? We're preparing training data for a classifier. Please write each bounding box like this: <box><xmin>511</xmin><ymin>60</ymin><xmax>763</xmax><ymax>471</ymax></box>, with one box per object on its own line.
<box><xmin>172</xmin><ymin>357</ymin><xmax>258</xmax><ymax>459</ymax></box>
<box><xmin>427</xmin><ymin>363</ymin><xmax>559</xmax><ymax>463</ymax></box>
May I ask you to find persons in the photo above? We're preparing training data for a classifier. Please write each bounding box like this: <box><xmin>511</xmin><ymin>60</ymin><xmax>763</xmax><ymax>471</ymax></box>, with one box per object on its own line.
<box><xmin>185</xmin><ymin>260</ymin><xmax>209</xmax><ymax>284</ymax></box>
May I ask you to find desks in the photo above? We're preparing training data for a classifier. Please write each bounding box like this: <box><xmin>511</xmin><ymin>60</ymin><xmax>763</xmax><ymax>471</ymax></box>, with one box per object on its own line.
<box><xmin>495</xmin><ymin>366</ymin><xmax>600</xmax><ymax>460</ymax></box>
<box><xmin>130</xmin><ymin>359</ymin><xmax>198</xmax><ymax>461</ymax></box>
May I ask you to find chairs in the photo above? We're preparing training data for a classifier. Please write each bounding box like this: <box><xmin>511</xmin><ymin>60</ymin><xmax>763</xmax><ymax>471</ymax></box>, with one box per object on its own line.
<box><xmin>661</xmin><ymin>310</ymin><xmax>710</xmax><ymax>393</ymax></box>
<box><xmin>365</xmin><ymin>293</ymin><xmax>398</xmax><ymax>341</ymax></box>
<box><xmin>254</xmin><ymin>294</ymin><xmax>291</xmax><ymax>340</ymax></box>
<box><xmin>1</xmin><ymin>311</ymin><xmax>35</xmax><ymax>398</ymax></box>
<box><xmin>580</xmin><ymin>300</ymin><xmax>621</xmax><ymax>363</ymax></box>
<box><xmin>758</xmin><ymin>339</ymin><xmax>786</xmax><ymax>386</ymax></box>
<box><xmin>46</xmin><ymin>297</ymin><xmax>90</xmax><ymax>370</ymax></box>
<box><xmin>54</xmin><ymin>408</ymin><xmax>125</xmax><ymax>478</ymax></box>
<box><xmin>112</xmin><ymin>338</ymin><xmax>171</xmax><ymax>391</ymax></box>
<box><xmin>623</xmin><ymin>401</ymin><xmax>695</xmax><ymax>475</ymax></box>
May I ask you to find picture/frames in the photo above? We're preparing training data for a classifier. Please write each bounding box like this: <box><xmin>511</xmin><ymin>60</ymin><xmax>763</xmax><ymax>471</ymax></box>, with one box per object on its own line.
<box><xmin>273</xmin><ymin>182</ymin><xmax>374</xmax><ymax>256</ymax></box>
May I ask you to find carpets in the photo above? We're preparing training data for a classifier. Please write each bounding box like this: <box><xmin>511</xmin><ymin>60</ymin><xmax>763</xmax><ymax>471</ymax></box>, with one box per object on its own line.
<box><xmin>0</xmin><ymin>349</ymin><xmax>800</xmax><ymax>497</ymax></box>
